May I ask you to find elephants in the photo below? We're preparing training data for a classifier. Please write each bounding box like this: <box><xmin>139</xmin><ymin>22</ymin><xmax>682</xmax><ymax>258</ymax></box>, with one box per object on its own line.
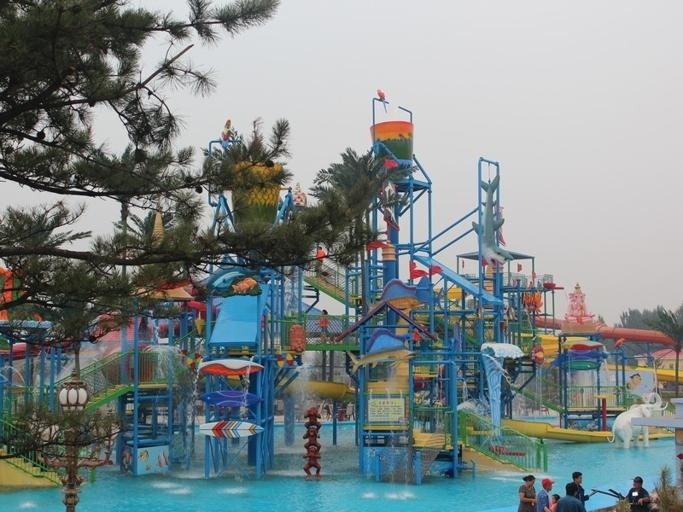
<box><xmin>606</xmin><ymin>392</ymin><xmax>669</xmax><ymax>449</ymax></box>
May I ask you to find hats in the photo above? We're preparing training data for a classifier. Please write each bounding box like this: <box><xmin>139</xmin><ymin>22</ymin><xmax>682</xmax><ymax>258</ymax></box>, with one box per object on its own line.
<box><xmin>632</xmin><ymin>476</ymin><xmax>643</xmax><ymax>483</ymax></box>
<box><xmin>542</xmin><ymin>479</ymin><xmax>554</xmax><ymax>486</ymax></box>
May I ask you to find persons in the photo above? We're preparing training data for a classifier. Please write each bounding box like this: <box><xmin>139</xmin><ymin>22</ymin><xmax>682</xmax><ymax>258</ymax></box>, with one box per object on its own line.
<box><xmin>536</xmin><ymin>281</ymin><xmax>541</xmax><ymax>287</ymax></box>
<box><xmin>315</xmin><ymin>245</ymin><xmax>325</xmax><ymax>276</ymax></box>
<box><xmin>318</xmin><ymin>309</ymin><xmax>329</xmax><ymax>342</ymax></box>
<box><xmin>302</xmin><ymin>406</ymin><xmax>321</xmax><ymax>480</ymax></box>
<box><xmin>517</xmin><ymin>471</ymin><xmax>589</xmax><ymax>511</ymax></box>
<box><xmin>625</xmin><ymin>373</ymin><xmax>642</xmax><ymax>391</ymax></box>
<box><xmin>528</xmin><ymin>281</ymin><xmax>533</xmax><ymax>288</ymax></box>
<box><xmin>625</xmin><ymin>475</ymin><xmax>650</xmax><ymax>511</ymax></box>
